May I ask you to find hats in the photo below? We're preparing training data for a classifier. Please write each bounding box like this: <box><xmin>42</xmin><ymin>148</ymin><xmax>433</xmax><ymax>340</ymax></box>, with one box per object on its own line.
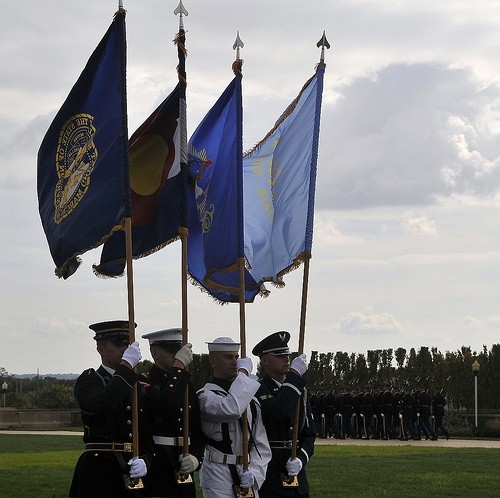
<box><xmin>251</xmin><ymin>331</ymin><xmax>292</xmax><ymax>356</ymax></box>
<box><xmin>88</xmin><ymin>320</ymin><xmax>138</xmax><ymax>341</ymax></box>
<box><xmin>205</xmin><ymin>336</ymin><xmax>241</xmax><ymax>352</ymax></box>
<box><xmin>141</xmin><ymin>327</ymin><xmax>189</xmax><ymax>346</ymax></box>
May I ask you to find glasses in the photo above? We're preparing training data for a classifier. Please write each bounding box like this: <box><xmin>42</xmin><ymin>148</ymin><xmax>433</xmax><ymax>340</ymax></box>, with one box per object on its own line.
<box><xmin>106</xmin><ymin>338</ymin><xmax>130</xmax><ymax>346</ymax></box>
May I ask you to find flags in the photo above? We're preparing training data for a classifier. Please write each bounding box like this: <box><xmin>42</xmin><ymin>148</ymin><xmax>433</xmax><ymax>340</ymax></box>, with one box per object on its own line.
<box><xmin>188</xmin><ymin>58</ymin><xmax>261</xmax><ymax>306</ymax></box>
<box><xmin>93</xmin><ymin>30</ymin><xmax>189</xmax><ymax>280</ymax></box>
<box><xmin>38</xmin><ymin>7</ymin><xmax>132</xmax><ymax>280</ymax></box>
<box><xmin>243</xmin><ymin>60</ymin><xmax>326</xmax><ymax>298</ymax></box>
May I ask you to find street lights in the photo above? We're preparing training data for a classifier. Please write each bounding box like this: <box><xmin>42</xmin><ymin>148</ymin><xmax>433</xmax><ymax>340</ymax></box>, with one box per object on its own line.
<box><xmin>471</xmin><ymin>359</ymin><xmax>480</xmax><ymax>427</ymax></box>
<box><xmin>2</xmin><ymin>381</ymin><xmax>8</xmax><ymax>408</ymax></box>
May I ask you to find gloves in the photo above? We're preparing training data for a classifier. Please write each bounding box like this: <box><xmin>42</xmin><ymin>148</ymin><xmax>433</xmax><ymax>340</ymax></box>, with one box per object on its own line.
<box><xmin>239</xmin><ymin>471</ymin><xmax>254</xmax><ymax>488</ymax></box>
<box><xmin>236</xmin><ymin>357</ymin><xmax>253</xmax><ymax>377</ymax></box>
<box><xmin>179</xmin><ymin>454</ymin><xmax>199</xmax><ymax>473</ymax></box>
<box><xmin>127</xmin><ymin>459</ymin><xmax>147</xmax><ymax>478</ymax></box>
<box><xmin>120</xmin><ymin>341</ymin><xmax>143</xmax><ymax>369</ymax></box>
<box><xmin>291</xmin><ymin>354</ymin><xmax>309</xmax><ymax>377</ymax></box>
<box><xmin>173</xmin><ymin>342</ymin><xmax>194</xmax><ymax>368</ymax></box>
<box><xmin>286</xmin><ymin>456</ymin><xmax>303</xmax><ymax>476</ymax></box>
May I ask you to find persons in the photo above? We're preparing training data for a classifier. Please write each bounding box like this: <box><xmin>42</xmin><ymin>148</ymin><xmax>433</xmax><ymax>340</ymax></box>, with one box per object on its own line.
<box><xmin>140</xmin><ymin>327</ymin><xmax>205</xmax><ymax>498</ymax></box>
<box><xmin>196</xmin><ymin>337</ymin><xmax>272</xmax><ymax>498</ymax></box>
<box><xmin>69</xmin><ymin>321</ymin><xmax>156</xmax><ymax>498</ymax></box>
<box><xmin>307</xmin><ymin>386</ymin><xmax>450</xmax><ymax>442</ymax></box>
<box><xmin>252</xmin><ymin>331</ymin><xmax>316</xmax><ymax>498</ymax></box>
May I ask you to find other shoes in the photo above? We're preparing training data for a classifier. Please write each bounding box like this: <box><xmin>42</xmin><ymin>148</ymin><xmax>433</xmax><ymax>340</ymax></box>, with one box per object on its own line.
<box><xmin>316</xmin><ymin>433</ymin><xmax>450</xmax><ymax>441</ymax></box>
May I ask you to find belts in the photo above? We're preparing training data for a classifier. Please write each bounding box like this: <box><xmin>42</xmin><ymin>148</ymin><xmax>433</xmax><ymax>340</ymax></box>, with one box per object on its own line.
<box><xmin>153</xmin><ymin>436</ymin><xmax>191</xmax><ymax>446</ymax></box>
<box><xmin>84</xmin><ymin>442</ymin><xmax>134</xmax><ymax>453</ymax></box>
<box><xmin>203</xmin><ymin>449</ymin><xmax>251</xmax><ymax>465</ymax></box>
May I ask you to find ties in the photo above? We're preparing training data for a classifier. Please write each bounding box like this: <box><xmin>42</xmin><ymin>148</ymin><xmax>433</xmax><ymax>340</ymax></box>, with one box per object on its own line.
<box><xmin>113</xmin><ymin>375</ymin><xmax>123</xmax><ymax>385</ymax></box>
